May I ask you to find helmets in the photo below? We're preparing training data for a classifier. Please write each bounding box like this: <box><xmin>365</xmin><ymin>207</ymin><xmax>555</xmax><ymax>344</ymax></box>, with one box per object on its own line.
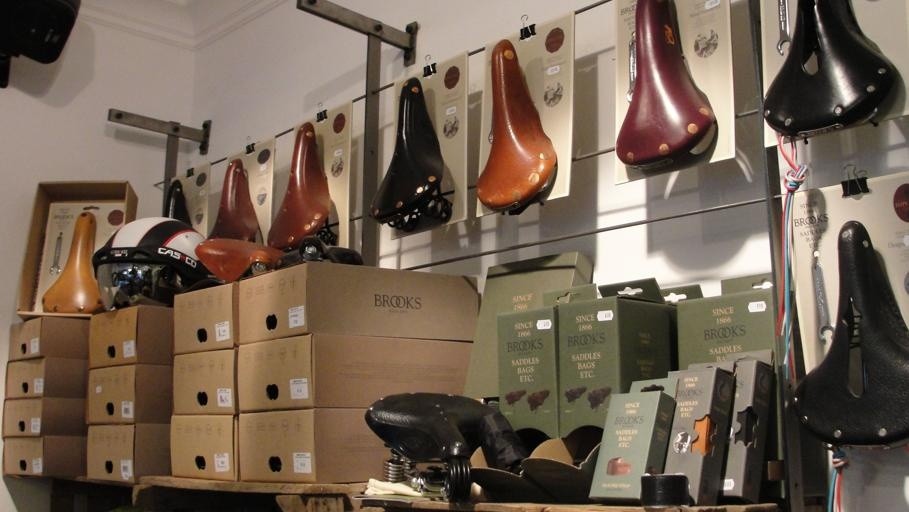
<box><xmin>92</xmin><ymin>217</ymin><xmax>207</xmax><ymax>311</ymax></box>
